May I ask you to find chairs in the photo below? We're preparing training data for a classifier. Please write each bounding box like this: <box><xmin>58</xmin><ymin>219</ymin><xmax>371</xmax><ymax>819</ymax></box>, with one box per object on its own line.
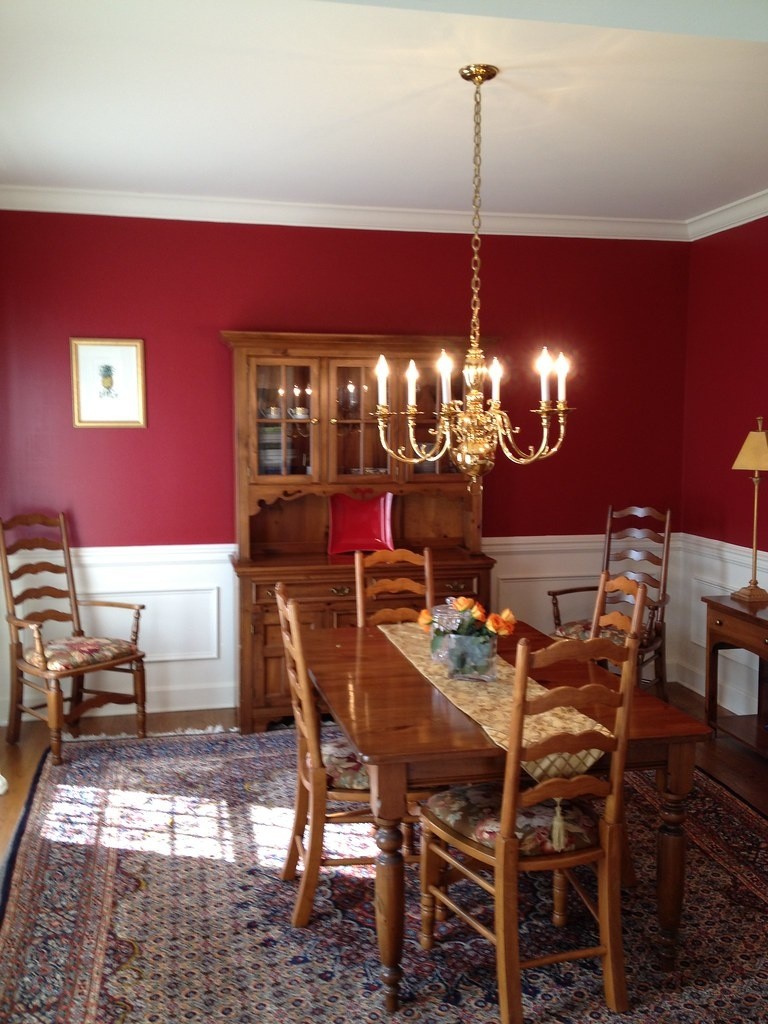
<box><xmin>419</xmin><ymin>630</ymin><xmax>640</xmax><ymax>1024</ymax></box>
<box><xmin>0</xmin><ymin>510</ymin><xmax>146</xmax><ymax>765</ymax></box>
<box><xmin>354</xmin><ymin>547</ymin><xmax>436</xmax><ymax>629</ymax></box>
<box><xmin>548</xmin><ymin>504</ymin><xmax>671</xmax><ymax>698</ymax></box>
<box><xmin>274</xmin><ymin>582</ymin><xmax>453</xmax><ymax>927</ymax></box>
<box><xmin>588</xmin><ymin>571</ymin><xmax>647</xmax><ymax>686</ymax></box>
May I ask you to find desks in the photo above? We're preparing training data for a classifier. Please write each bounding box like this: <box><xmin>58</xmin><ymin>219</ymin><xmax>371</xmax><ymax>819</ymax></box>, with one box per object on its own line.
<box><xmin>296</xmin><ymin>618</ymin><xmax>715</xmax><ymax>1013</ymax></box>
<box><xmin>700</xmin><ymin>596</ymin><xmax>768</xmax><ymax>760</ymax></box>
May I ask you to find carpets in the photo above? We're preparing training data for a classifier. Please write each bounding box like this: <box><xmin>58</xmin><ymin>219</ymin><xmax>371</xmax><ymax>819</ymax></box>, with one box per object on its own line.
<box><xmin>0</xmin><ymin>723</ymin><xmax>768</xmax><ymax>1024</ymax></box>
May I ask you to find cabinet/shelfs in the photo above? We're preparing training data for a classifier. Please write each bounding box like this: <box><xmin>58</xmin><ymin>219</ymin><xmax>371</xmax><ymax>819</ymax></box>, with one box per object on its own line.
<box><xmin>220</xmin><ymin>330</ymin><xmax>489</xmax><ymax>734</ymax></box>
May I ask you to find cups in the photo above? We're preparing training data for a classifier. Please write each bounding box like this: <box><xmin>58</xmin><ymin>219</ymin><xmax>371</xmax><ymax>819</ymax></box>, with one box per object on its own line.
<box><xmin>287</xmin><ymin>407</ymin><xmax>309</xmax><ymax>420</ymax></box>
<box><xmin>260</xmin><ymin>406</ymin><xmax>280</xmax><ymax>419</ymax></box>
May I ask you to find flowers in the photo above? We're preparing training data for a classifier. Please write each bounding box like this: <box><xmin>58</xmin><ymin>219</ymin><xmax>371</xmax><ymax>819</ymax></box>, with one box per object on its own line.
<box><xmin>418</xmin><ymin>597</ymin><xmax>516</xmax><ymax>635</ymax></box>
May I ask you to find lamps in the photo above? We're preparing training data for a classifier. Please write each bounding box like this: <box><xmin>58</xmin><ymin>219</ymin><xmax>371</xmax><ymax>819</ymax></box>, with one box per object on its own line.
<box><xmin>731</xmin><ymin>414</ymin><xmax>768</xmax><ymax>603</ymax></box>
<box><xmin>372</xmin><ymin>64</ymin><xmax>578</xmax><ymax>495</ymax></box>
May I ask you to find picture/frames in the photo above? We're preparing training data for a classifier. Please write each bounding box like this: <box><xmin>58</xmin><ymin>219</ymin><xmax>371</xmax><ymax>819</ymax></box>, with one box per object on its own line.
<box><xmin>69</xmin><ymin>337</ymin><xmax>146</xmax><ymax>429</ymax></box>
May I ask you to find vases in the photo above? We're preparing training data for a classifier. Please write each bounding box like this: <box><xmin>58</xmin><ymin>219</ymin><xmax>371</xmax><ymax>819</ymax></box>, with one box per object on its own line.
<box><xmin>431</xmin><ymin>635</ymin><xmax>496</xmax><ymax>681</ymax></box>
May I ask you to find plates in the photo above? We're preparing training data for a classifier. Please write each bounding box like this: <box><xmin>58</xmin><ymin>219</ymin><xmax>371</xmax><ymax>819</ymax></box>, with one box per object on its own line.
<box><xmin>259</xmin><ymin>427</ymin><xmax>293</xmax><ymax>474</ymax></box>
<box><xmin>414</xmin><ymin>444</ymin><xmax>435</xmax><ymax>473</ymax></box>
